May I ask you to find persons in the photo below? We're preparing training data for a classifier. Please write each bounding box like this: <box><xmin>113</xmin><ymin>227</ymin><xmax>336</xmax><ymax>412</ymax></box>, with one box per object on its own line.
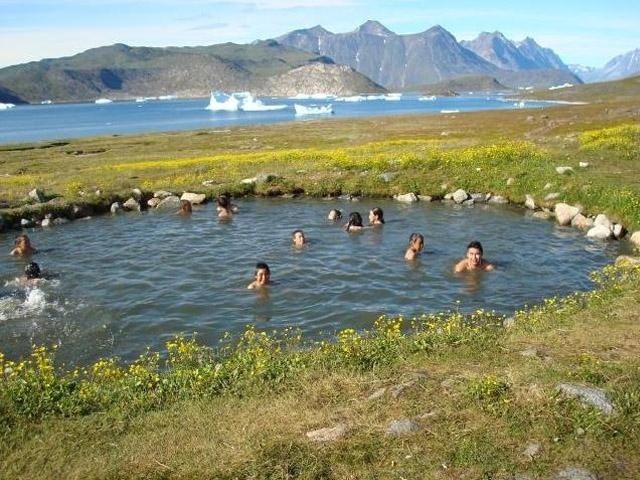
<box><xmin>19</xmin><ymin>262</ymin><xmax>45</xmax><ymax>288</ymax></box>
<box><xmin>179</xmin><ymin>200</ymin><xmax>192</xmax><ymax>213</ymax></box>
<box><xmin>9</xmin><ymin>235</ymin><xmax>38</xmax><ymax>256</ymax></box>
<box><xmin>404</xmin><ymin>233</ymin><xmax>425</xmax><ymax>259</ymax></box>
<box><xmin>329</xmin><ymin>209</ymin><xmax>341</xmax><ymax>219</ymax></box>
<box><xmin>456</xmin><ymin>241</ymin><xmax>494</xmax><ymax>272</ymax></box>
<box><xmin>293</xmin><ymin>230</ymin><xmax>305</xmax><ymax>245</ymax></box>
<box><xmin>368</xmin><ymin>207</ymin><xmax>384</xmax><ymax>224</ymax></box>
<box><xmin>247</xmin><ymin>262</ymin><xmax>271</xmax><ymax>289</ymax></box>
<box><xmin>216</xmin><ymin>192</ymin><xmax>238</xmax><ymax>217</ymax></box>
<box><xmin>345</xmin><ymin>212</ymin><xmax>362</xmax><ymax>229</ymax></box>
